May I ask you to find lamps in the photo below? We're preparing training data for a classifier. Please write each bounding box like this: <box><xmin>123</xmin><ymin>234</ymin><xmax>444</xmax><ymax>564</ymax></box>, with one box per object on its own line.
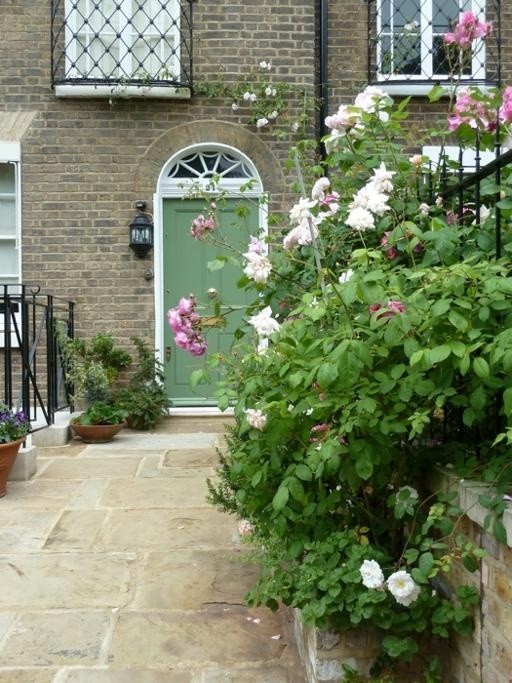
<box><xmin>128</xmin><ymin>201</ymin><xmax>153</xmax><ymax>258</ymax></box>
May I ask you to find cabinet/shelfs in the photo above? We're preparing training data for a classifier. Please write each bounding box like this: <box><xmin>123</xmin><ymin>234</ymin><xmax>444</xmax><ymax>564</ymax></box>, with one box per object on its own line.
<box><xmin>0</xmin><ymin>141</ymin><xmax>22</xmax><ymax>347</ymax></box>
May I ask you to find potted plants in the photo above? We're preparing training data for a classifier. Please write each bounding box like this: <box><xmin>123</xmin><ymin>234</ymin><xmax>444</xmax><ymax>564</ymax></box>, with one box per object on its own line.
<box><xmin>57</xmin><ymin>319</ymin><xmax>172</xmax><ymax>443</ymax></box>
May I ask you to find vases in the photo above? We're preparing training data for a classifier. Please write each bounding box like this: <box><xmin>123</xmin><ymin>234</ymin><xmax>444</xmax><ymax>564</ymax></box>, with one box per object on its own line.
<box><xmin>0</xmin><ymin>436</ymin><xmax>27</xmax><ymax>497</ymax></box>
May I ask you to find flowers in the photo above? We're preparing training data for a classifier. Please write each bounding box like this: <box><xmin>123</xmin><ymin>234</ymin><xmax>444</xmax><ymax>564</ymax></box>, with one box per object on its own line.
<box><xmin>0</xmin><ymin>401</ymin><xmax>31</xmax><ymax>444</ymax></box>
